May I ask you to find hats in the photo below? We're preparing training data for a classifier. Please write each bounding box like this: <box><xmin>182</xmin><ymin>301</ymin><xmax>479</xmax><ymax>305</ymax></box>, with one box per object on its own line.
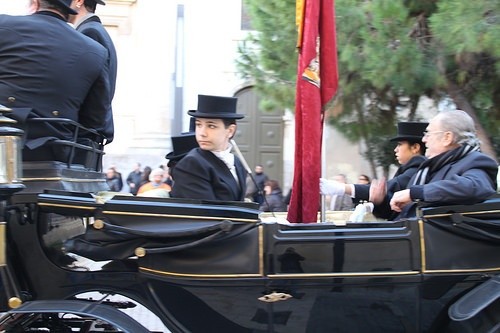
<box><xmin>58</xmin><ymin>0</ymin><xmax>79</xmax><ymax>15</ymax></box>
<box><xmin>95</xmin><ymin>0</ymin><xmax>105</xmax><ymax>6</ymax></box>
<box><xmin>148</xmin><ymin>168</ymin><xmax>168</xmax><ymax>182</ymax></box>
<box><xmin>388</xmin><ymin>121</ymin><xmax>429</xmax><ymax>142</ymax></box>
<box><xmin>186</xmin><ymin>94</ymin><xmax>245</xmax><ymax>119</ymax></box>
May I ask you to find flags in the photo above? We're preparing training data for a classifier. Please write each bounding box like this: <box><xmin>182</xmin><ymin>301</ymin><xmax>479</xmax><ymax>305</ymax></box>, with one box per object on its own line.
<box><xmin>286</xmin><ymin>0</ymin><xmax>339</xmax><ymax>223</ymax></box>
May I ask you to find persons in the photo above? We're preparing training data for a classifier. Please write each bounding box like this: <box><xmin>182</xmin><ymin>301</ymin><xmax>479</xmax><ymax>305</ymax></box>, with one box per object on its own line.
<box><xmin>0</xmin><ymin>0</ymin><xmax>498</xmax><ymax>220</ymax></box>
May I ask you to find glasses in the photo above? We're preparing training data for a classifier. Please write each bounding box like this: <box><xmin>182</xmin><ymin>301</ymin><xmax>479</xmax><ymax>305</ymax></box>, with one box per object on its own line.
<box><xmin>422</xmin><ymin>131</ymin><xmax>445</xmax><ymax>137</ymax></box>
<box><xmin>358</xmin><ymin>179</ymin><xmax>367</xmax><ymax>181</ymax></box>
